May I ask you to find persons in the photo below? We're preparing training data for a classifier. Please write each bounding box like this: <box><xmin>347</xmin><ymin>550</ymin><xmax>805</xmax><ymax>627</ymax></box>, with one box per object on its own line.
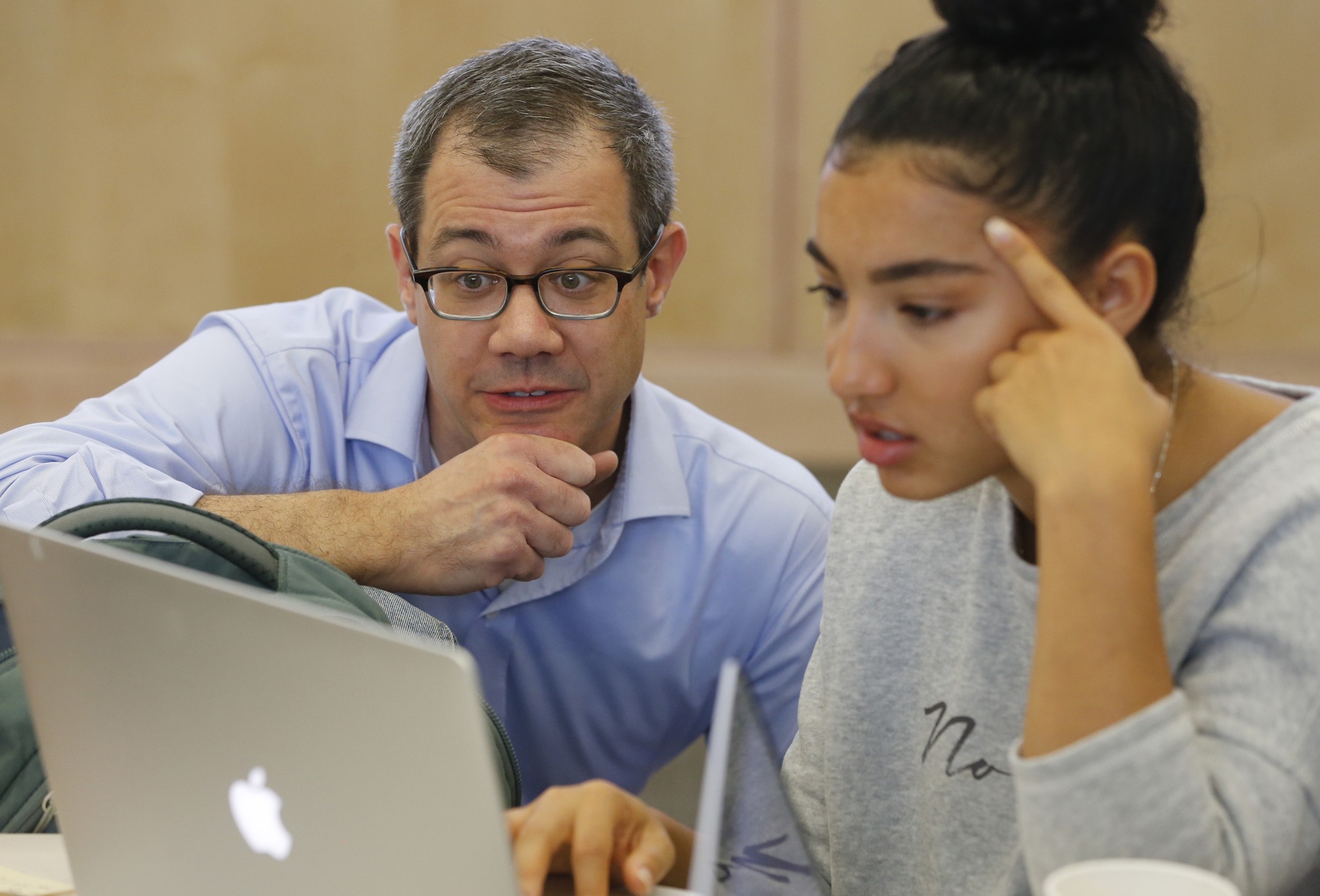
<box><xmin>505</xmin><ymin>0</ymin><xmax>1320</xmax><ymax>896</ymax></box>
<box><xmin>1</xmin><ymin>38</ymin><xmax>834</xmax><ymax>811</ymax></box>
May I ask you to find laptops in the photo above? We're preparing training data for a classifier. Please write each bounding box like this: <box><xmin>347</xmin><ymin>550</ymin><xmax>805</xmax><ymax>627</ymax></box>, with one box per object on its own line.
<box><xmin>693</xmin><ymin>661</ymin><xmax>832</xmax><ymax>896</ymax></box>
<box><xmin>0</xmin><ymin>521</ymin><xmax>697</xmax><ymax>896</ymax></box>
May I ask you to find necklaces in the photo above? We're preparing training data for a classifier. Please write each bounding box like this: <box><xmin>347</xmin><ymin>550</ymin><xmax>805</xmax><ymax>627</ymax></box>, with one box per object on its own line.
<box><xmin>1150</xmin><ymin>337</ymin><xmax>1179</xmax><ymax>494</ymax></box>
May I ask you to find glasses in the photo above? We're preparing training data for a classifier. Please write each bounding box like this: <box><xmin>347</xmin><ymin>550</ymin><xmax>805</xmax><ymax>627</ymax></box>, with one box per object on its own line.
<box><xmin>399</xmin><ymin>222</ymin><xmax>665</xmax><ymax>320</ymax></box>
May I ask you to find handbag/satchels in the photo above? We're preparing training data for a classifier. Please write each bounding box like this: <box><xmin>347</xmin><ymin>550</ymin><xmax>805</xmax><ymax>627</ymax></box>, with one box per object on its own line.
<box><xmin>0</xmin><ymin>496</ymin><xmax>522</xmax><ymax>836</ymax></box>
